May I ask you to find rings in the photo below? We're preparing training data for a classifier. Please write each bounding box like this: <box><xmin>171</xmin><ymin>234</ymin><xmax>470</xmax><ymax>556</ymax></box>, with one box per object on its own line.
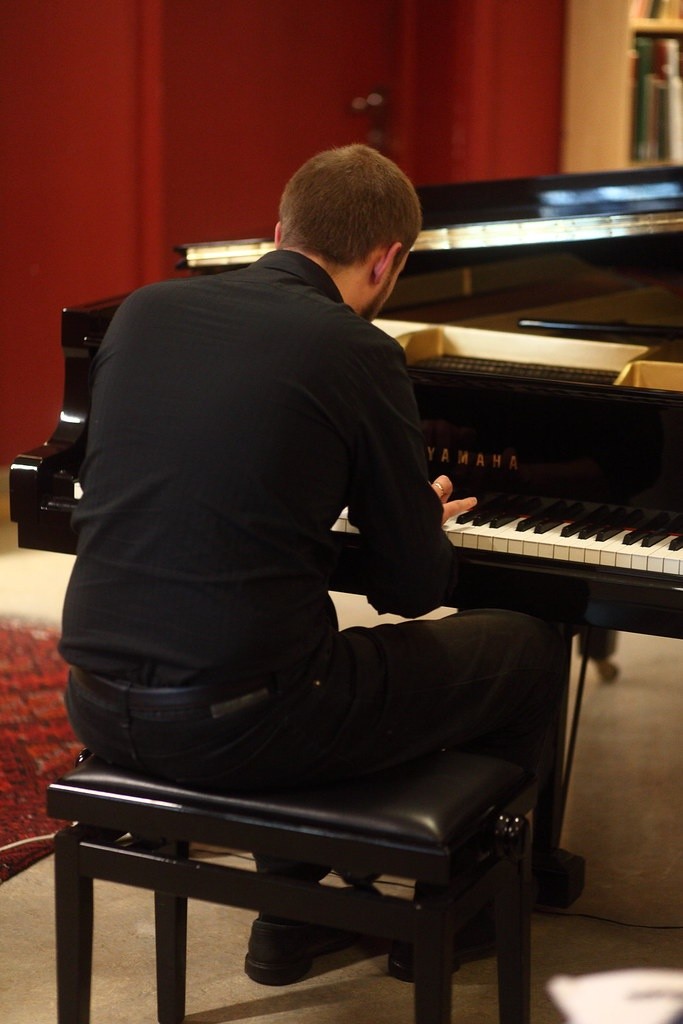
<box><xmin>434</xmin><ymin>481</ymin><xmax>444</xmax><ymax>497</ymax></box>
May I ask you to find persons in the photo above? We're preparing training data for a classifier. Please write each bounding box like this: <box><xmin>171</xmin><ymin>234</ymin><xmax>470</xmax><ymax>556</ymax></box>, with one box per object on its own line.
<box><xmin>54</xmin><ymin>141</ymin><xmax>563</xmax><ymax>988</ymax></box>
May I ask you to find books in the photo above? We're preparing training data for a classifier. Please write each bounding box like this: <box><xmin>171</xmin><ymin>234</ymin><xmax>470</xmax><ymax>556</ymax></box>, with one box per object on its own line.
<box><xmin>632</xmin><ymin>0</ymin><xmax>683</xmax><ymax>165</ymax></box>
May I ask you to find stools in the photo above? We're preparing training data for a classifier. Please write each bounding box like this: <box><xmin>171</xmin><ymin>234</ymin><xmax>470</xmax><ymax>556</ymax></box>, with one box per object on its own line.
<box><xmin>46</xmin><ymin>752</ymin><xmax>540</xmax><ymax>1023</ymax></box>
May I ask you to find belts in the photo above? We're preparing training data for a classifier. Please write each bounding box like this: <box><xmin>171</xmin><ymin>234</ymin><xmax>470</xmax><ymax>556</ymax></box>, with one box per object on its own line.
<box><xmin>71</xmin><ymin>665</ymin><xmax>262</xmax><ymax>711</ymax></box>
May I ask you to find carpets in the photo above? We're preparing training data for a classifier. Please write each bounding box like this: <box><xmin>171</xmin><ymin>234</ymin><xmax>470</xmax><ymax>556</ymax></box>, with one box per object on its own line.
<box><xmin>0</xmin><ymin>614</ymin><xmax>85</xmax><ymax>885</ymax></box>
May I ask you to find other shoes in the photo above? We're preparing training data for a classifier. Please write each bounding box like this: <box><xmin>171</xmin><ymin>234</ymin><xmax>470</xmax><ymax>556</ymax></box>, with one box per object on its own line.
<box><xmin>245</xmin><ymin>885</ymin><xmax>384</xmax><ymax>986</ymax></box>
<box><xmin>387</xmin><ymin>913</ymin><xmax>496</xmax><ymax>983</ymax></box>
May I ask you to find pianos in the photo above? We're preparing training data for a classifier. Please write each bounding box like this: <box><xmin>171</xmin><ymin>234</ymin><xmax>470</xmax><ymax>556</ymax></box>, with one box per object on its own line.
<box><xmin>7</xmin><ymin>166</ymin><xmax>683</xmax><ymax>642</ymax></box>
